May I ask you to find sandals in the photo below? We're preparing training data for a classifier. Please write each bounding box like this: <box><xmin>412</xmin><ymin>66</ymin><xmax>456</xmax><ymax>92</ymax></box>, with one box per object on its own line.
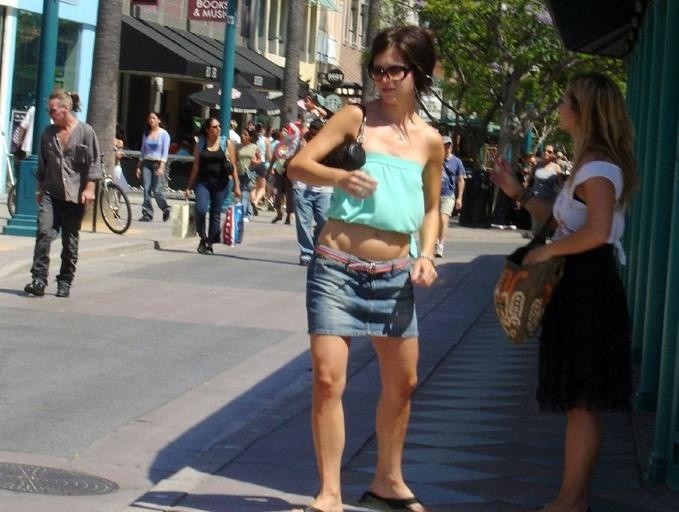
<box><xmin>251</xmin><ymin>196</ymin><xmax>276</xmax><ymax>215</ymax></box>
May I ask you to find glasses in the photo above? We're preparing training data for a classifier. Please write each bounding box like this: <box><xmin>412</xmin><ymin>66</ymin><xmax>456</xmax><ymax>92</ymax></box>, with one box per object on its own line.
<box><xmin>543</xmin><ymin>148</ymin><xmax>553</xmax><ymax>154</ymax></box>
<box><xmin>372</xmin><ymin>62</ymin><xmax>418</xmax><ymax>82</ymax></box>
<box><xmin>208</xmin><ymin>125</ymin><xmax>222</xmax><ymax>129</ymax></box>
<box><xmin>47</xmin><ymin>107</ymin><xmax>62</xmax><ymax>113</ymax></box>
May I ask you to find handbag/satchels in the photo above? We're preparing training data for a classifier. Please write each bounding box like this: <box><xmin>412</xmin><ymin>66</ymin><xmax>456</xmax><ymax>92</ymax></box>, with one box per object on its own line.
<box><xmin>224</xmin><ymin>196</ymin><xmax>244</xmax><ymax>245</ymax></box>
<box><xmin>493</xmin><ymin>205</ymin><xmax>566</xmax><ymax>344</ymax></box>
<box><xmin>319</xmin><ymin>103</ymin><xmax>369</xmax><ymax>170</ymax></box>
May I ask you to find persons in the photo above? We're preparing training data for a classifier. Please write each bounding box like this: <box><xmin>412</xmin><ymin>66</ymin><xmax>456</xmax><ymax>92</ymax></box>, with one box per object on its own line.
<box><xmin>524</xmin><ymin>143</ymin><xmax>563</xmax><ymax>240</ymax></box>
<box><xmin>14</xmin><ymin>103</ymin><xmax>36</xmax><ymax>178</ymax></box>
<box><xmin>269</xmin><ymin>130</ymin><xmax>279</xmax><ymax>148</ymax></box>
<box><xmin>184</xmin><ymin>118</ymin><xmax>242</xmax><ymax>256</ymax></box>
<box><xmin>245</xmin><ymin>120</ymin><xmax>259</xmax><ymax>145</ymax></box>
<box><xmin>507</xmin><ymin>164</ymin><xmax>525</xmax><ymax>227</ymax></box>
<box><xmin>23</xmin><ymin>91</ymin><xmax>103</xmax><ymax>298</ymax></box>
<box><xmin>266</xmin><ymin>125</ymin><xmax>297</xmax><ymax>225</ymax></box>
<box><xmin>112</xmin><ymin>122</ymin><xmax>129</xmax><ymax>219</ymax></box>
<box><xmin>287</xmin><ymin>25</ymin><xmax>446</xmax><ymax>512</ymax></box>
<box><xmin>555</xmin><ymin>151</ymin><xmax>571</xmax><ymax>174</ymax></box>
<box><xmin>486</xmin><ymin>73</ymin><xmax>641</xmax><ymax>512</ymax></box>
<box><xmin>229</xmin><ymin>118</ymin><xmax>241</xmax><ymax>146</ymax></box>
<box><xmin>235</xmin><ymin>129</ymin><xmax>263</xmax><ymax>219</ymax></box>
<box><xmin>135</xmin><ymin>112</ymin><xmax>173</xmax><ymax>223</ymax></box>
<box><xmin>523</xmin><ymin>156</ymin><xmax>537</xmax><ymax>188</ymax></box>
<box><xmin>301</xmin><ymin>94</ymin><xmax>328</xmax><ymax>129</ymax></box>
<box><xmin>289</xmin><ymin>118</ymin><xmax>336</xmax><ymax>267</ymax></box>
<box><xmin>433</xmin><ymin>136</ymin><xmax>467</xmax><ymax>261</ymax></box>
<box><xmin>252</xmin><ymin>123</ymin><xmax>272</xmax><ymax>217</ymax></box>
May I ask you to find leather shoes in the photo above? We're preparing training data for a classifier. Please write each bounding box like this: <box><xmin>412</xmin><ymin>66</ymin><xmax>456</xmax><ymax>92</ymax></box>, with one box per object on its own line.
<box><xmin>24</xmin><ymin>279</ymin><xmax>45</xmax><ymax>296</ymax></box>
<box><xmin>285</xmin><ymin>216</ymin><xmax>290</xmax><ymax>224</ymax></box>
<box><xmin>197</xmin><ymin>238</ymin><xmax>213</xmax><ymax>255</ymax></box>
<box><xmin>139</xmin><ymin>214</ymin><xmax>153</xmax><ymax>222</ymax></box>
<box><xmin>162</xmin><ymin>210</ymin><xmax>169</xmax><ymax>221</ymax></box>
<box><xmin>57</xmin><ymin>280</ymin><xmax>70</xmax><ymax>297</ymax></box>
<box><xmin>270</xmin><ymin>214</ymin><xmax>283</xmax><ymax>224</ymax></box>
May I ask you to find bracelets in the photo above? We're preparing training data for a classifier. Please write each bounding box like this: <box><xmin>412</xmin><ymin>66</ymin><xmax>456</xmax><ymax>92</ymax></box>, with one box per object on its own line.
<box><xmin>420</xmin><ymin>252</ymin><xmax>439</xmax><ymax>263</ymax></box>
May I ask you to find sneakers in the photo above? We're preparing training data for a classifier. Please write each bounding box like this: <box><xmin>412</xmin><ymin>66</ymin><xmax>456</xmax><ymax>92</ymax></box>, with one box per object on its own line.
<box><xmin>434</xmin><ymin>242</ymin><xmax>443</xmax><ymax>257</ymax></box>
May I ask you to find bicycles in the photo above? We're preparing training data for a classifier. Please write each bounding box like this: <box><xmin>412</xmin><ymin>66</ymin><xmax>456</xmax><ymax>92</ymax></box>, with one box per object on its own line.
<box><xmin>8</xmin><ymin>147</ymin><xmax>131</xmax><ymax>233</ymax></box>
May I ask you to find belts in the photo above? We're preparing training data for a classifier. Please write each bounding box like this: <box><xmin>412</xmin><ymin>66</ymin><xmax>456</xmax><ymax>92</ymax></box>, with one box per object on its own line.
<box><xmin>315</xmin><ymin>245</ymin><xmax>409</xmax><ymax>273</ymax></box>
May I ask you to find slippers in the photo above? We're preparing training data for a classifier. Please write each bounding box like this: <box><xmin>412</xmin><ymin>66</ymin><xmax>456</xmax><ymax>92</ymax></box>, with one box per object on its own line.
<box><xmin>360</xmin><ymin>492</ymin><xmax>428</xmax><ymax>512</ymax></box>
<box><xmin>292</xmin><ymin>504</ymin><xmax>324</xmax><ymax>512</ymax></box>
